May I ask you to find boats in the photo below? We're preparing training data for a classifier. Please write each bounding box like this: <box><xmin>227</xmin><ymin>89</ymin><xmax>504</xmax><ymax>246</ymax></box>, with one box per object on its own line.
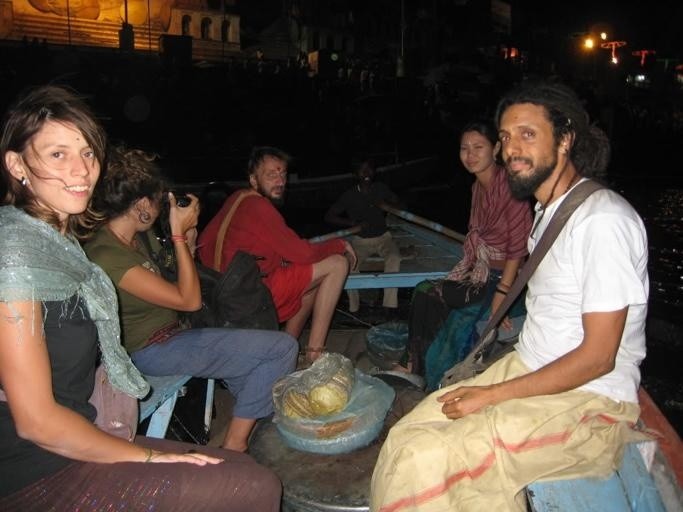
<box><xmin>164</xmin><ymin>199</ymin><xmax>682</xmax><ymax>512</ymax></box>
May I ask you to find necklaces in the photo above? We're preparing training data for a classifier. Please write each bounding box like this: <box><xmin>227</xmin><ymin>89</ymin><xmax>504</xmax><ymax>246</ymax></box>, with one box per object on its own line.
<box><xmin>109</xmin><ymin>224</ymin><xmax>132</xmax><ymax>244</ymax></box>
<box><xmin>537</xmin><ymin>171</ymin><xmax>580</xmax><ymax>211</ymax></box>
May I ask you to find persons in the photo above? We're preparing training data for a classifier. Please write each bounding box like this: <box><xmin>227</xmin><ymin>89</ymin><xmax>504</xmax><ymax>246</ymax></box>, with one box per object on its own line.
<box><xmin>196</xmin><ymin>144</ymin><xmax>360</xmax><ymax>370</ymax></box>
<box><xmin>392</xmin><ymin>118</ymin><xmax>534</xmax><ymax>379</ymax></box>
<box><xmin>369</xmin><ymin>80</ymin><xmax>650</xmax><ymax>510</ymax></box>
<box><xmin>1</xmin><ymin>84</ymin><xmax>284</xmax><ymax>510</ymax></box>
<box><xmin>324</xmin><ymin>159</ymin><xmax>402</xmax><ymax>317</ymax></box>
<box><xmin>83</xmin><ymin>147</ymin><xmax>301</xmax><ymax>455</ymax></box>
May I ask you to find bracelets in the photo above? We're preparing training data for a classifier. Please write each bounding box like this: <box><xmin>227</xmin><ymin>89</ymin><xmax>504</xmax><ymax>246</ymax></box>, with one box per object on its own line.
<box><xmin>144</xmin><ymin>446</ymin><xmax>153</xmax><ymax>462</ymax></box>
<box><xmin>171</xmin><ymin>234</ymin><xmax>185</xmax><ymax>242</ymax></box>
<box><xmin>495</xmin><ymin>280</ymin><xmax>511</xmax><ymax>296</ymax></box>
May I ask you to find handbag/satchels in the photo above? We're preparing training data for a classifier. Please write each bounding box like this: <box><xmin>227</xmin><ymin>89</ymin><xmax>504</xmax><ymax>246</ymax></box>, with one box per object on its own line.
<box><xmin>90</xmin><ymin>364</ymin><xmax>139</xmax><ymax>444</ymax></box>
<box><xmin>195</xmin><ymin>250</ymin><xmax>280</xmax><ymax>329</ymax></box>
<box><xmin>439</xmin><ymin>357</ymin><xmax>490</xmax><ymax>391</ymax></box>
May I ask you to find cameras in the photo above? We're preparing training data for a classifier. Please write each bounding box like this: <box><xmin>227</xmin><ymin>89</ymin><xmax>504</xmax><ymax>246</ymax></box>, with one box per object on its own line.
<box><xmin>158</xmin><ymin>191</ymin><xmax>203</xmax><ymax>244</ymax></box>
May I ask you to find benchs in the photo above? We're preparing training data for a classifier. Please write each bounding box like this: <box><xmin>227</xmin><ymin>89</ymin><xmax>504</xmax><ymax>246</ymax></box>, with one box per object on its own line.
<box><xmin>137</xmin><ymin>271</ymin><xmax>665</xmax><ymax>512</ymax></box>
